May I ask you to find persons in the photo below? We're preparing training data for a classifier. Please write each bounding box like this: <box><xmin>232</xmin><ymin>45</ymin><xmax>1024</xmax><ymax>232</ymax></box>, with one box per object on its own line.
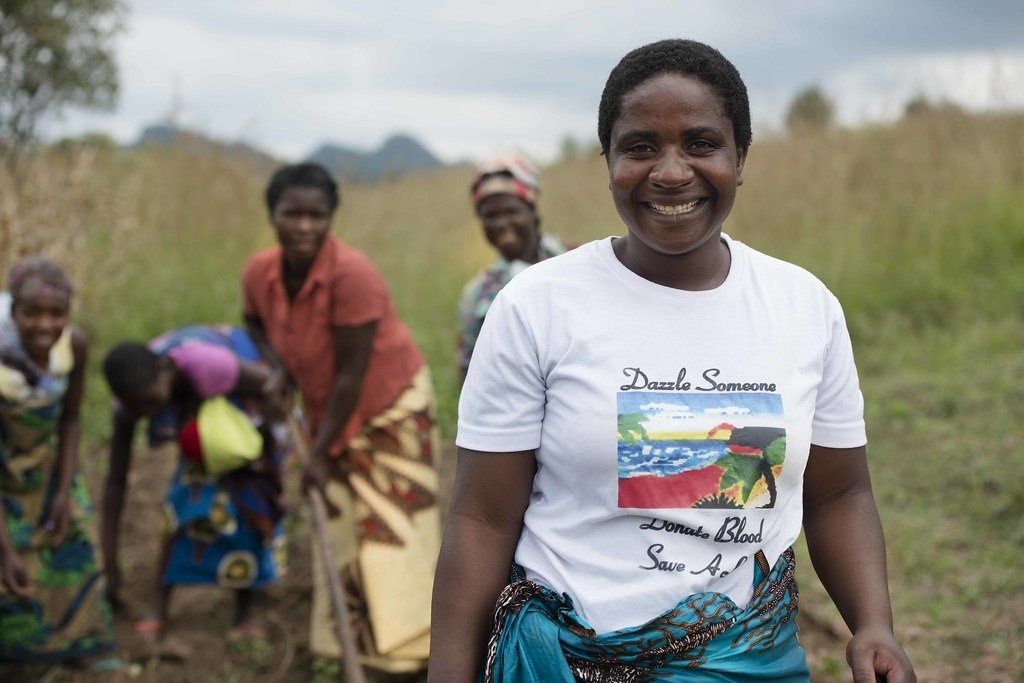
<box><xmin>244</xmin><ymin>164</ymin><xmax>444</xmax><ymax>683</ymax></box>
<box><xmin>431</xmin><ymin>43</ymin><xmax>918</xmax><ymax>683</ymax></box>
<box><xmin>453</xmin><ymin>155</ymin><xmax>586</xmax><ymax>405</ymax></box>
<box><xmin>0</xmin><ymin>259</ymin><xmax>142</xmax><ymax>677</ymax></box>
<box><xmin>98</xmin><ymin>323</ymin><xmax>289</xmax><ymax>647</ymax></box>
